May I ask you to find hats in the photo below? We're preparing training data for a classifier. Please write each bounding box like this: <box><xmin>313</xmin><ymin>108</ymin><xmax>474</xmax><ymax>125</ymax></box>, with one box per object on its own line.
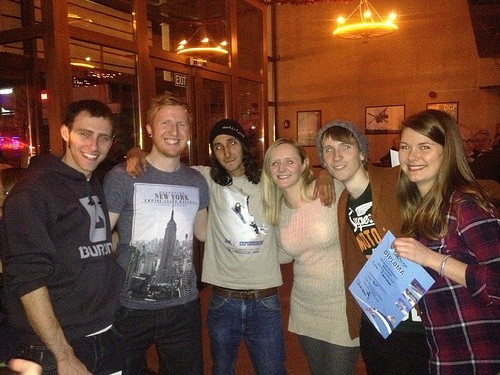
<box><xmin>317</xmin><ymin>119</ymin><xmax>367</xmax><ymax>164</ymax></box>
<box><xmin>209</xmin><ymin>118</ymin><xmax>247</xmax><ymax>144</ymax></box>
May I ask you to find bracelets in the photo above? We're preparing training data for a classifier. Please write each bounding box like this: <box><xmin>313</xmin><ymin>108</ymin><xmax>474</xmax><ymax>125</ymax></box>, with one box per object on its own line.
<box><xmin>437</xmin><ymin>254</ymin><xmax>452</xmax><ymax>278</ymax></box>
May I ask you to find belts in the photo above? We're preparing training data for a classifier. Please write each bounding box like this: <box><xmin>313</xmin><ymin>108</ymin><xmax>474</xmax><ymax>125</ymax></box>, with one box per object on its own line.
<box><xmin>213</xmin><ymin>286</ymin><xmax>278</xmax><ymax>300</ymax></box>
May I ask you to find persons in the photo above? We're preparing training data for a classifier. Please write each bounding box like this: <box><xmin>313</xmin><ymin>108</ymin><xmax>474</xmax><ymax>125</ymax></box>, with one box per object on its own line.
<box><xmin>0</xmin><ymin>100</ymin><xmax>118</xmax><ymax>375</ymax></box>
<box><xmin>391</xmin><ymin>108</ymin><xmax>500</xmax><ymax>375</ymax></box>
<box><xmin>124</xmin><ymin>119</ymin><xmax>337</xmax><ymax>375</ymax></box>
<box><xmin>316</xmin><ymin>119</ymin><xmax>500</xmax><ymax>375</ymax></box>
<box><xmin>261</xmin><ymin>137</ymin><xmax>361</xmax><ymax>375</ymax></box>
<box><xmin>101</xmin><ymin>93</ymin><xmax>210</xmax><ymax>375</ymax></box>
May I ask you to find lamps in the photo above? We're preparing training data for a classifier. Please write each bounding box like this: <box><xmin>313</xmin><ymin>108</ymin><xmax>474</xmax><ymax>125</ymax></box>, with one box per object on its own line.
<box><xmin>334</xmin><ymin>0</ymin><xmax>398</xmax><ymax>43</ymax></box>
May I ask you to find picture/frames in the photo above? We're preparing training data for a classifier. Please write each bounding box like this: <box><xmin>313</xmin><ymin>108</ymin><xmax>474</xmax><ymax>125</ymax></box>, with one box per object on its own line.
<box><xmin>427</xmin><ymin>102</ymin><xmax>458</xmax><ymax>123</ymax></box>
<box><xmin>296</xmin><ymin>110</ymin><xmax>322</xmax><ymax>147</ymax></box>
<box><xmin>365</xmin><ymin>105</ymin><xmax>406</xmax><ymax>134</ymax></box>
